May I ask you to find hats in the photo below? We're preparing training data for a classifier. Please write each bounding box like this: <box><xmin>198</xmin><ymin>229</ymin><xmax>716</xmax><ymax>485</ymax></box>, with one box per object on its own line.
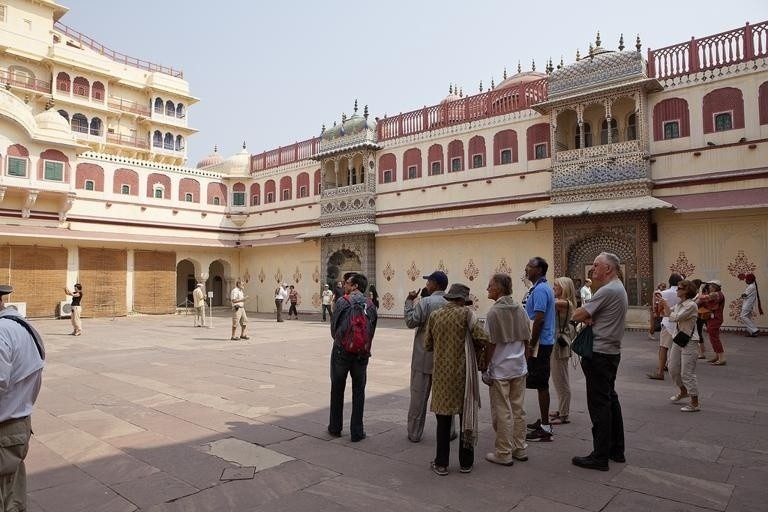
<box><xmin>422</xmin><ymin>271</ymin><xmax>450</xmax><ymax>289</ymax></box>
<box><xmin>707</xmin><ymin>279</ymin><xmax>724</xmax><ymax>288</ymax></box>
<box><xmin>444</xmin><ymin>284</ymin><xmax>473</xmax><ymax>305</ymax></box>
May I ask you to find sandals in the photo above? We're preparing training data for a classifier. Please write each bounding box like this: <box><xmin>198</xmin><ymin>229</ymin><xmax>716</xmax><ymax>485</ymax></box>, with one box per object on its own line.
<box><xmin>550</xmin><ymin>411</ymin><xmax>571</xmax><ymax>426</ymax></box>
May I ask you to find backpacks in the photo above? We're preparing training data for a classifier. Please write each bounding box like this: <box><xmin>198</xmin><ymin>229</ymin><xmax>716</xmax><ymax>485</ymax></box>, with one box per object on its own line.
<box><xmin>340</xmin><ymin>296</ymin><xmax>375</xmax><ymax>359</ymax></box>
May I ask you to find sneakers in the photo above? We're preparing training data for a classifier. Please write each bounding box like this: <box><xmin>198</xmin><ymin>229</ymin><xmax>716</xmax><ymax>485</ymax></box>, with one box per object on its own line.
<box><xmin>571</xmin><ymin>450</ymin><xmax>609</xmax><ymax>472</ymax></box>
<box><xmin>350</xmin><ymin>431</ymin><xmax>368</xmax><ymax>444</ymax></box>
<box><xmin>458</xmin><ymin>462</ymin><xmax>474</xmax><ymax>474</ymax></box>
<box><xmin>647</xmin><ymin>371</ymin><xmax>666</xmax><ymax>380</ymax></box>
<box><xmin>680</xmin><ymin>400</ymin><xmax>704</xmax><ymax>414</ymax></box>
<box><xmin>229</xmin><ymin>334</ymin><xmax>250</xmax><ymax>343</ymax></box>
<box><xmin>327</xmin><ymin>424</ymin><xmax>343</xmax><ymax>438</ymax></box>
<box><xmin>708</xmin><ymin>356</ymin><xmax>728</xmax><ymax>367</ymax></box>
<box><xmin>429</xmin><ymin>457</ymin><xmax>450</xmax><ymax>476</ymax></box>
<box><xmin>669</xmin><ymin>390</ymin><xmax>690</xmax><ymax>403</ymax></box>
<box><xmin>526</xmin><ymin>419</ymin><xmax>555</xmax><ymax>445</ymax></box>
<box><xmin>488</xmin><ymin>447</ymin><xmax>530</xmax><ymax>469</ymax></box>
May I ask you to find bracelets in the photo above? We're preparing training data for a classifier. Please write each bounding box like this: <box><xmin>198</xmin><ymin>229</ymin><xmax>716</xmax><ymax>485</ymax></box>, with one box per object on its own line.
<box><xmin>528</xmin><ymin>346</ymin><xmax>534</xmax><ymax>349</ymax></box>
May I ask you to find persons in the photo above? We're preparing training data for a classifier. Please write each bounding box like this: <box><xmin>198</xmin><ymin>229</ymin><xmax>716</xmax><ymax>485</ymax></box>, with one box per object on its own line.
<box><xmin>274</xmin><ymin>273</ymin><xmax>379</xmax><ymax>323</ymax></box>
<box><xmin>63</xmin><ymin>283</ymin><xmax>82</xmax><ymax>336</ymax></box>
<box><xmin>192</xmin><ymin>282</ymin><xmax>207</xmax><ymax>327</ymax></box>
<box><xmin>404</xmin><ymin>271</ymin><xmax>457</xmax><ymax>442</ymax></box>
<box><xmin>572</xmin><ymin>251</ymin><xmax>629</xmax><ymax>471</ymax></box>
<box><xmin>328</xmin><ymin>273</ymin><xmax>378</xmax><ymax>441</ymax></box>
<box><xmin>230</xmin><ymin>280</ymin><xmax>249</xmax><ymax>340</ymax></box>
<box><xmin>422</xmin><ymin>283</ymin><xmax>489</xmax><ymax>476</ymax></box>
<box><xmin>481</xmin><ymin>275</ymin><xmax>531</xmax><ymax>467</ymax></box>
<box><xmin>645</xmin><ymin>272</ymin><xmax>764</xmax><ymax>412</ymax></box>
<box><xmin>521</xmin><ymin>256</ymin><xmax>556</xmax><ymax>443</ymax></box>
<box><xmin>1</xmin><ymin>286</ymin><xmax>48</xmax><ymax>511</ymax></box>
<box><xmin>580</xmin><ymin>278</ymin><xmax>592</xmax><ymax>306</ymax></box>
<box><xmin>549</xmin><ymin>276</ymin><xmax>578</xmax><ymax>424</ymax></box>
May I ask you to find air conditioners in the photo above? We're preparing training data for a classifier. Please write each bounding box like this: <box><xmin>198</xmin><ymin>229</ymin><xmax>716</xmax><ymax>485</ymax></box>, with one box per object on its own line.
<box><xmin>60</xmin><ymin>301</ymin><xmax>72</xmax><ymax>320</ymax></box>
<box><xmin>4</xmin><ymin>303</ymin><xmax>26</xmax><ymax>317</ymax></box>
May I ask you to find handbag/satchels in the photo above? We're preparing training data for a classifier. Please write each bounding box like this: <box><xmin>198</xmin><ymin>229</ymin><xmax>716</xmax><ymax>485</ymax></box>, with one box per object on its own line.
<box><xmin>671</xmin><ymin>328</ymin><xmax>693</xmax><ymax>347</ymax></box>
<box><xmin>567</xmin><ymin>325</ymin><xmax>593</xmax><ymax>354</ymax></box>
